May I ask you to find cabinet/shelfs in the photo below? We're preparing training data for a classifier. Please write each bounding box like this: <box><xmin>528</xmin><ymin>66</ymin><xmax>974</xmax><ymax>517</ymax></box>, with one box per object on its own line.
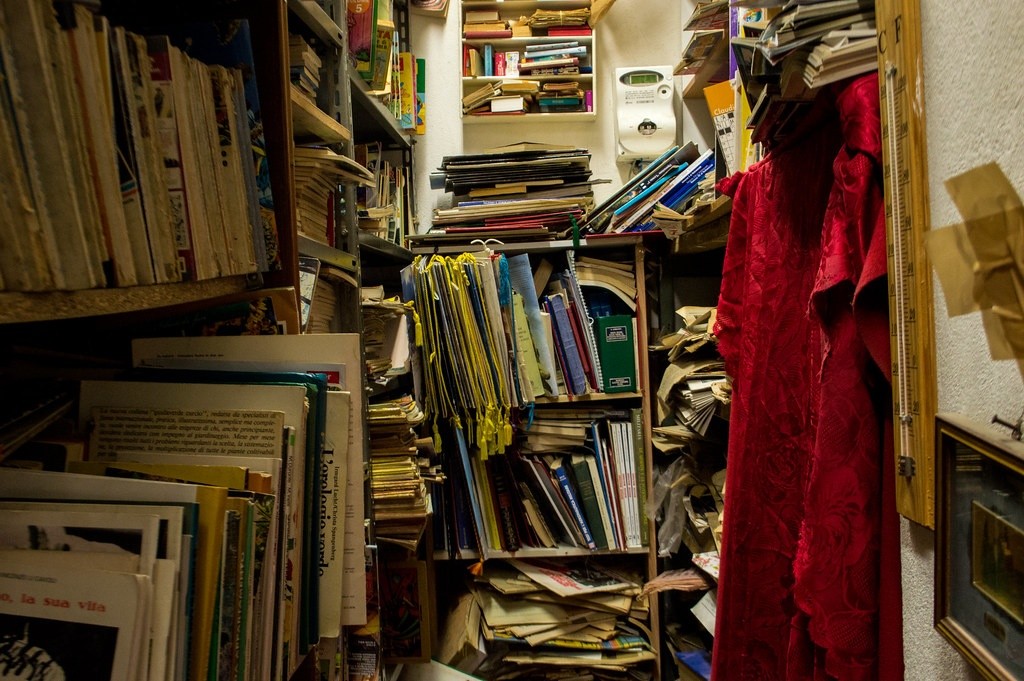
<box><xmin>659</xmin><ymin>211</ymin><xmax>731</xmax><ymax>681</ymax></box>
<box><xmin>0</xmin><ymin>0</ymin><xmax>419</xmax><ymax>681</ymax></box>
<box><xmin>458</xmin><ymin>0</ymin><xmax>597</xmax><ymax>123</ymax></box>
<box><xmin>405</xmin><ymin>235</ymin><xmax>663</xmax><ymax>681</ymax></box>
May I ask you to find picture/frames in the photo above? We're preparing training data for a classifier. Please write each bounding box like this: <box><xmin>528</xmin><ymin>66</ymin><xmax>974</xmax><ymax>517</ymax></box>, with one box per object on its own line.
<box><xmin>932</xmin><ymin>414</ymin><xmax>1024</xmax><ymax>681</ymax></box>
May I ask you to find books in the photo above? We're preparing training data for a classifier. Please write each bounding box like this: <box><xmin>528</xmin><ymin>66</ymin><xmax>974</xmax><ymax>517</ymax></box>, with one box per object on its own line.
<box><xmin>0</xmin><ymin>0</ymin><xmax>881</xmax><ymax>681</ymax></box>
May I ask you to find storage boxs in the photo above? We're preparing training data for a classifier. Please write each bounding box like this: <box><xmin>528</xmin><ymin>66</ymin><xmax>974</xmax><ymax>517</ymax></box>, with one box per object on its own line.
<box><xmin>595</xmin><ymin>314</ymin><xmax>636</xmax><ymax>393</ymax></box>
